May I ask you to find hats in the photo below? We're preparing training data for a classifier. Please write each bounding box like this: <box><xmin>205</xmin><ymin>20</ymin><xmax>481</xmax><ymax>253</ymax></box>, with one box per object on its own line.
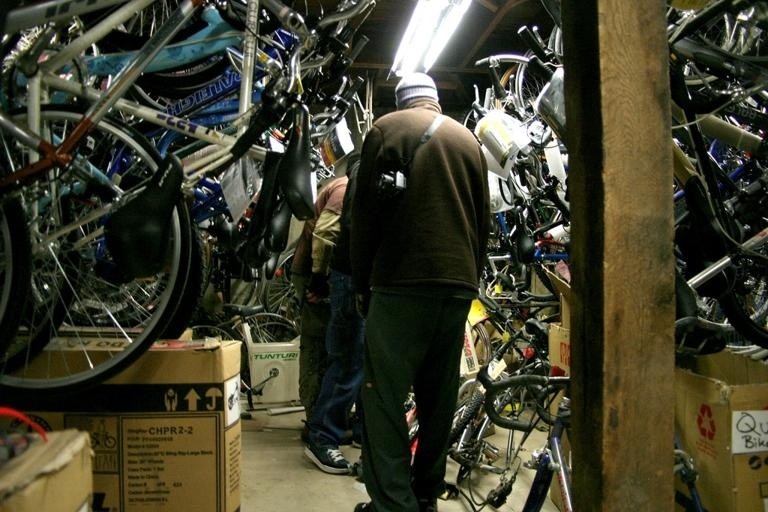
<box><xmin>394</xmin><ymin>72</ymin><xmax>440</xmax><ymax>107</ymax></box>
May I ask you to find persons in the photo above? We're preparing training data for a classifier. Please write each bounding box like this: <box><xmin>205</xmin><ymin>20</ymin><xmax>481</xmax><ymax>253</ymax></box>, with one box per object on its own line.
<box><xmin>288</xmin><ymin>74</ymin><xmax>490</xmax><ymax>511</ymax></box>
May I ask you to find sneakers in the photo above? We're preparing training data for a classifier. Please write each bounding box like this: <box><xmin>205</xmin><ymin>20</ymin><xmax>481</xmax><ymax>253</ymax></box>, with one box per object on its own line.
<box><xmin>303</xmin><ymin>441</ymin><xmax>353</xmax><ymax>475</ymax></box>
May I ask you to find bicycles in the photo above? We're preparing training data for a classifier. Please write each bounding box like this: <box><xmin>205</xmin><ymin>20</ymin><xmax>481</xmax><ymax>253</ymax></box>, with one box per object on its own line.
<box><xmin>409</xmin><ymin>0</ymin><xmax>768</xmax><ymax>512</ymax></box>
<box><xmin>0</xmin><ymin>0</ymin><xmax>376</xmax><ymax>402</ymax></box>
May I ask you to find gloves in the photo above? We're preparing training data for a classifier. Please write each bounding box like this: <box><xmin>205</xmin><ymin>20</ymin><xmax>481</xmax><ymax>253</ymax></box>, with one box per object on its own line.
<box><xmin>308</xmin><ymin>273</ymin><xmax>330</xmax><ymax>299</ymax></box>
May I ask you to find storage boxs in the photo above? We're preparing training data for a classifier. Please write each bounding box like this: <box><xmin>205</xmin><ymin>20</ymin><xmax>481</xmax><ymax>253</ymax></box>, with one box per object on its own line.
<box><xmin>547</xmin><ymin>265</ymin><xmax>768</xmax><ymax>512</ymax></box>
<box><xmin>0</xmin><ymin>337</ymin><xmax>241</xmax><ymax>509</ymax></box>
